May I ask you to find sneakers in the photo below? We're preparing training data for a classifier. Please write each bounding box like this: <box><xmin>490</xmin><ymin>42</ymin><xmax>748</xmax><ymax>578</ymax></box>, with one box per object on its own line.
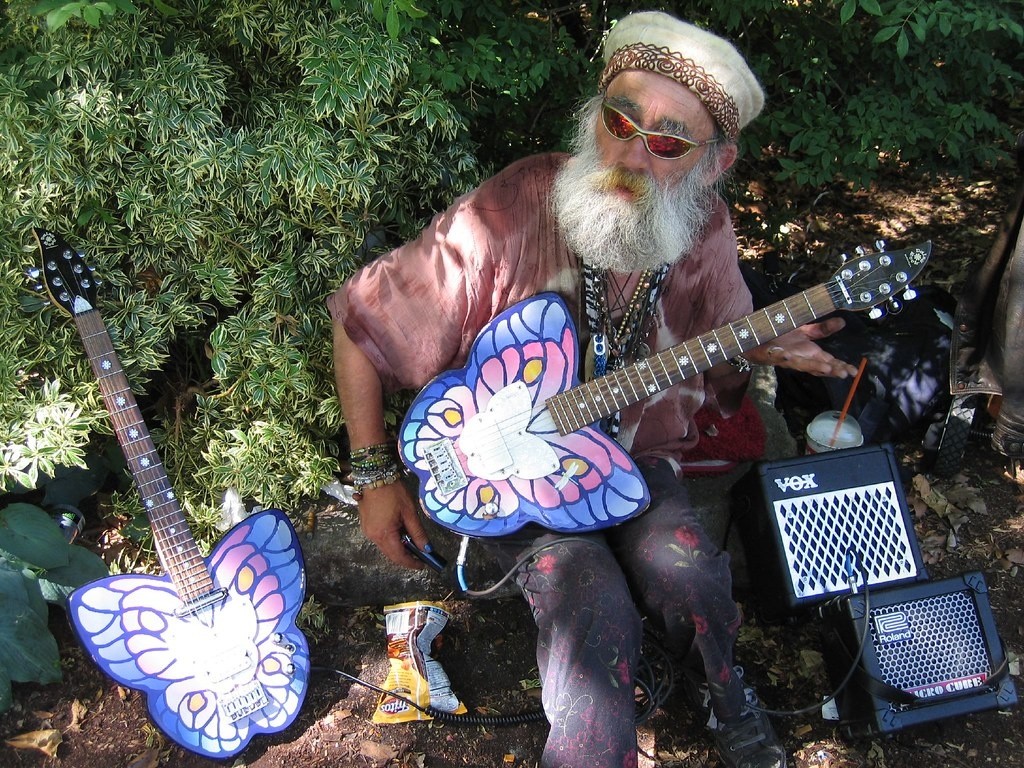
<box><xmin>693</xmin><ymin>665</ymin><xmax>788</xmax><ymax>768</ymax></box>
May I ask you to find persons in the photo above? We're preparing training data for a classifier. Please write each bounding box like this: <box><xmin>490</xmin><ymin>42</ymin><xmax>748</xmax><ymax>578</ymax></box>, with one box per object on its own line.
<box><xmin>325</xmin><ymin>11</ymin><xmax>857</xmax><ymax>767</ymax></box>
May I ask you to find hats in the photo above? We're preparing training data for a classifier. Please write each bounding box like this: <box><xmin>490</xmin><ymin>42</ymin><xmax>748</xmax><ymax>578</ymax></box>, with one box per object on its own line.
<box><xmin>597</xmin><ymin>11</ymin><xmax>764</xmax><ymax>144</ymax></box>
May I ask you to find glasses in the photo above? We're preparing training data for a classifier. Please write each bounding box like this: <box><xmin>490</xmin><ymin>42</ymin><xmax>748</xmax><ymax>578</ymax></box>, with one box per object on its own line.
<box><xmin>601</xmin><ymin>98</ymin><xmax>720</xmax><ymax>160</ymax></box>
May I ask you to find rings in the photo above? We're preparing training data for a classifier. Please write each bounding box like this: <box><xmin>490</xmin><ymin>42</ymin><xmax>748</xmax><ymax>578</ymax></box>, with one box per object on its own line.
<box><xmin>769</xmin><ymin>347</ymin><xmax>784</xmax><ymax>357</ymax></box>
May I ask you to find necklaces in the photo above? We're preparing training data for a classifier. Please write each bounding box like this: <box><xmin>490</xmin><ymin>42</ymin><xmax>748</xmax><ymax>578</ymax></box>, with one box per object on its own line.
<box><xmin>578</xmin><ymin>259</ymin><xmax>670</xmax><ymax>439</ymax></box>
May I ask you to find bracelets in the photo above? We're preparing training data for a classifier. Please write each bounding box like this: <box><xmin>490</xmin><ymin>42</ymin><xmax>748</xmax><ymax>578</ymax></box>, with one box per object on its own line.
<box><xmin>349</xmin><ymin>443</ymin><xmax>401</xmax><ymax>501</ymax></box>
<box><xmin>728</xmin><ymin>355</ymin><xmax>753</xmax><ymax>374</ymax></box>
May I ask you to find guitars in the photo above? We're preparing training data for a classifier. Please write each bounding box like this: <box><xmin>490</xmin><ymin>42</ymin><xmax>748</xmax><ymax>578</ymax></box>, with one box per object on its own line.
<box><xmin>398</xmin><ymin>237</ymin><xmax>938</xmax><ymax>539</ymax></box>
<box><xmin>26</xmin><ymin>221</ymin><xmax>310</xmax><ymax>756</ymax></box>
<box><xmin>313</xmin><ymin>489</ymin><xmax>313</xmax><ymax>491</ymax></box>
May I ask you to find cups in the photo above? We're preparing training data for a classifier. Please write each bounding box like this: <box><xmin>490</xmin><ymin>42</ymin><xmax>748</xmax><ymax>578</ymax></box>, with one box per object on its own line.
<box><xmin>805</xmin><ymin>411</ymin><xmax>864</xmax><ymax>457</ymax></box>
